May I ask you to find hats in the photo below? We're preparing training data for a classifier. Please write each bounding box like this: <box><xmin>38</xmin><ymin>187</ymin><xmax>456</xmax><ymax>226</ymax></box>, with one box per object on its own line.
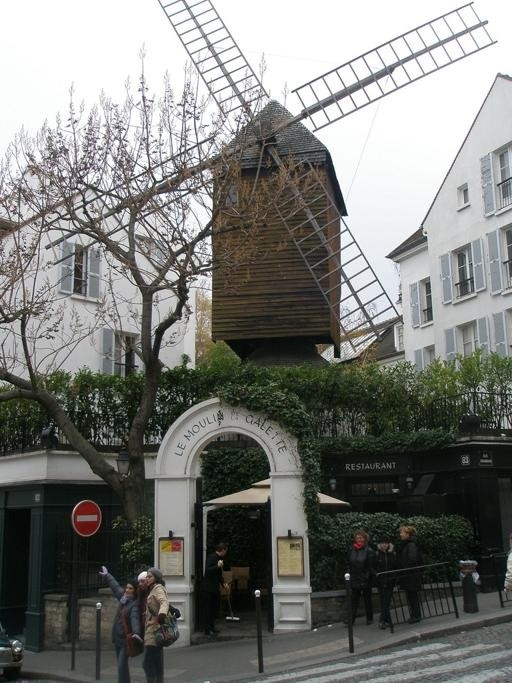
<box><xmin>379</xmin><ymin>533</ymin><xmax>390</xmax><ymax>542</ymax></box>
<box><xmin>126</xmin><ymin>567</ymin><xmax>163</xmax><ymax>590</ymax></box>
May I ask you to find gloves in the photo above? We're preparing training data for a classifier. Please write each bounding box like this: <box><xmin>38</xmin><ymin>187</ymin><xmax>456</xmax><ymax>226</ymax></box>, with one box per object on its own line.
<box><xmin>217</xmin><ymin>559</ymin><xmax>224</xmax><ymax>567</ymax></box>
<box><xmin>223</xmin><ymin>582</ymin><xmax>230</xmax><ymax>589</ymax></box>
<box><xmin>99</xmin><ymin>565</ymin><xmax>108</xmax><ymax>576</ymax></box>
<box><xmin>157</xmin><ymin>613</ymin><xmax>166</xmax><ymax>625</ymax></box>
<box><xmin>171</xmin><ymin>607</ymin><xmax>181</xmax><ymax>618</ymax></box>
<box><xmin>132</xmin><ymin>634</ymin><xmax>142</xmax><ymax>641</ymax></box>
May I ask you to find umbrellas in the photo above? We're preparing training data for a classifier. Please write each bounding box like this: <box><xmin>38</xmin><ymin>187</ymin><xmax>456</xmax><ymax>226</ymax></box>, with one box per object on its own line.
<box><xmin>201</xmin><ymin>478</ymin><xmax>350</xmax><ymax>576</ymax></box>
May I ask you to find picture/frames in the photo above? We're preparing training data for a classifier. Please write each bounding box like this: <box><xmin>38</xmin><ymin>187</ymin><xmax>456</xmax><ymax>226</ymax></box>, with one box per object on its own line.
<box><xmin>158</xmin><ymin>537</ymin><xmax>184</xmax><ymax>577</ymax></box>
<box><xmin>276</xmin><ymin>536</ymin><xmax>304</xmax><ymax>578</ymax></box>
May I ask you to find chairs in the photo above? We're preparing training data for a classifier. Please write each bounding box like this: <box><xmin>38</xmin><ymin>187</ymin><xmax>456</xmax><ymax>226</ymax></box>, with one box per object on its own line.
<box><xmin>223</xmin><ymin>567</ymin><xmax>249</xmax><ymax>592</ymax></box>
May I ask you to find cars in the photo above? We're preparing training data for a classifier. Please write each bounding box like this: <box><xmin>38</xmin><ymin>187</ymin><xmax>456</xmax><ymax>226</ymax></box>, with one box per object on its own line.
<box><xmin>1</xmin><ymin>624</ymin><xmax>24</xmax><ymax>681</ymax></box>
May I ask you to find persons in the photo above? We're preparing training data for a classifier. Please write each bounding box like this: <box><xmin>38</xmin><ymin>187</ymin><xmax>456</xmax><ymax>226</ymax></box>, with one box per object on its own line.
<box><xmin>503</xmin><ymin>533</ymin><xmax>512</xmax><ymax>593</ymax></box>
<box><xmin>99</xmin><ymin>566</ymin><xmax>143</xmax><ymax>683</ymax></box>
<box><xmin>343</xmin><ymin>529</ymin><xmax>378</xmax><ymax>625</ymax></box>
<box><xmin>397</xmin><ymin>526</ymin><xmax>422</xmax><ymax>622</ymax></box>
<box><xmin>375</xmin><ymin>535</ymin><xmax>399</xmax><ymax>628</ymax></box>
<box><xmin>201</xmin><ymin>542</ymin><xmax>230</xmax><ymax>636</ymax></box>
<box><xmin>138</xmin><ymin>571</ymin><xmax>181</xmax><ymax>634</ymax></box>
<box><xmin>143</xmin><ymin>568</ymin><xmax>169</xmax><ymax>683</ymax></box>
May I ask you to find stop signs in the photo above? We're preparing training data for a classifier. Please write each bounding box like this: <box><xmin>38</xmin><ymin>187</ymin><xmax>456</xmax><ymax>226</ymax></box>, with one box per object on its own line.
<box><xmin>72</xmin><ymin>501</ymin><xmax>101</xmax><ymax>536</ymax></box>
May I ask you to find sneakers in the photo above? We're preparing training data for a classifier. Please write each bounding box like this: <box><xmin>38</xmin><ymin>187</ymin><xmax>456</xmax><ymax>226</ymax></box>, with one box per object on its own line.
<box><xmin>205</xmin><ymin>624</ymin><xmax>221</xmax><ymax>635</ymax></box>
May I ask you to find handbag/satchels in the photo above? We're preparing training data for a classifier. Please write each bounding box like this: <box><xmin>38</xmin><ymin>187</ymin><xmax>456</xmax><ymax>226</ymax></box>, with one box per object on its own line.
<box><xmin>126</xmin><ymin>633</ymin><xmax>144</xmax><ymax>656</ymax></box>
<box><xmin>153</xmin><ymin>611</ymin><xmax>180</xmax><ymax>648</ymax></box>
